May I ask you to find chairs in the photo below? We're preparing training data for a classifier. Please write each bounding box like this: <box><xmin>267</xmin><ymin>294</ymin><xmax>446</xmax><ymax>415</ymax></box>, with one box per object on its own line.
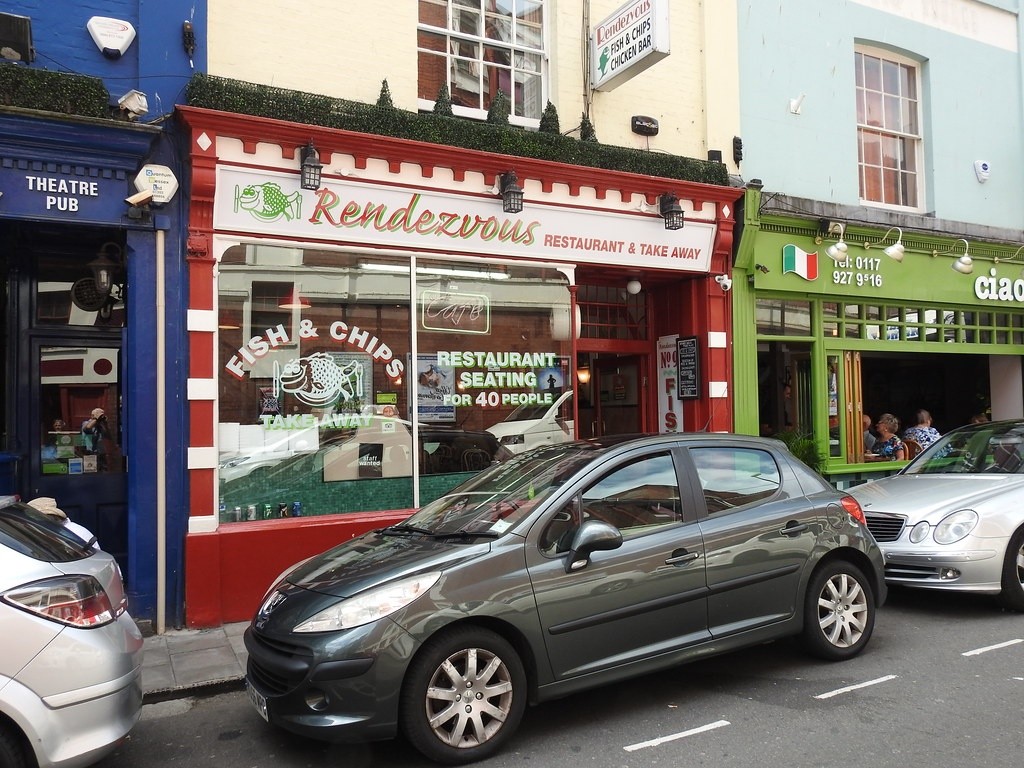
<box><xmin>584</xmin><ymin>469</ymin><xmax>677</xmax><ymax>530</ymax></box>
<box><xmin>902</xmin><ymin>440</ymin><xmax>923</xmax><ymax>460</ymax></box>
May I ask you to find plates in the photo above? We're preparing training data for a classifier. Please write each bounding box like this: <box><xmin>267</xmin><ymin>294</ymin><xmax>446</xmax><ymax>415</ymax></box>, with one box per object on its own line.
<box><xmin>865</xmin><ymin>454</ymin><xmax>879</xmax><ymax>457</ymax></box>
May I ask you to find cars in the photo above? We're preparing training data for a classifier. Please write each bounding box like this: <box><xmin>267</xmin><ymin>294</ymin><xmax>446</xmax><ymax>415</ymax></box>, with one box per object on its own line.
<box><xmin>215</xmin><ymin>387</ymin><xmax>575</xmax><ymax>522</ymax></box>
<box><xmin>0</xmin><ymin>494</ymin><xmax>147</xmax><ymax>768</ymax></box>
<box><xmin>839</xmin><ymin>418</ymin><xmax>1024</xmax><ymax>615</ymax></box>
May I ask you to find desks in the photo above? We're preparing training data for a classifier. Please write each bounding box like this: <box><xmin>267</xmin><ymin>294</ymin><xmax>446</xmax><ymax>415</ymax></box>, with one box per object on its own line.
<box><xmin>864</xmin><ymin>456</ymin><xmax>890</xmax><ymax>463</ymax></box>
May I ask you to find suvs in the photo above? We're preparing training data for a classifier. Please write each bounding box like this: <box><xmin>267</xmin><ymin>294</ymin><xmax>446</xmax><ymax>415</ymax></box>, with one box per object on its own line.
<box><xmin>243</xmin><ymin>431</ymin><xmax>889</xmax><ymax>766</ymax></box>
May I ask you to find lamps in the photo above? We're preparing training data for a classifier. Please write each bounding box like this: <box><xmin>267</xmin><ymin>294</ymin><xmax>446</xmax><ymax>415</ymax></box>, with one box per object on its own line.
<box><xmin>118</xmin><ymin>89</ymin><xmax>148</xmax><ymax>121</ymax></box>
<box><xmin>627</xmin><ymin>276</ymin><xmax>641</xmax><ymax>294</ymax></box>
<box><xmin>815</xmin><ymin>223</ymin><xmax>847</xmax><ymax>262</ymax></box>
<box><xmin>660</xmin><ymin>190</ymin><xmax>685</xmax><ymax>230</ymax></box>
<box><xmin>932</xmin><ymin>238</ymin><xmax>973</xmax><ymax>274</ymax></box>
<box><xmin>500</xmin><ymin>168</ymin><xmax>525</xmax><ymax>213</ymax></box>
<box><xmin>219</xmin><ymin>310</ymin><xmax>239</xmax><ymax>329</ymax></box>
<box><xmin>356</xmin><ymin>258</ymin><xmax>513</xmax><ymax>280</ymax></box>
<box><xmin>864</xmin><ymin>227</ymin><xmax>904</xmax><ymax>263</ymax></box>
<box><xmin>87</xmin><ymin>241</ymin><xmax>127</xmax><ymax>300</ymax></box>
<box><xmin>300</xmin><ymin>137</ymin><xmax>323</xmax><ymax>191</ymax></box>
<box><xmin>279</xmin><ymin>287</ymin><xmax>311</xmax><ymax>309</ymax></box>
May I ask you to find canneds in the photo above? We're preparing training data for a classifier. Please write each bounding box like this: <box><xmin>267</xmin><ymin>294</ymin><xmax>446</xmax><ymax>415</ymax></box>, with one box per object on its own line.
<box><xmin>232</xmin><ymin>506</ymin><xmax>241</xmax><ymax>522</ymax></box>
<box><xmin>278</xmin><ymin>502</ymin><xmax>288</xmax><ymax>518</ymax></box>
<box><xmin>292</xmin><ymin>501</ymin><xmax>302</xmax><ymax>517</ymax></box>
<box><xmin>247</xmin><ymin>505</ymin><xmax>256</xmax><ymax>521</ymax></box>
<box><xmin>263</xmin><ymin>503</ymin><xmax>272</xmax><ymax>520</ymax></box>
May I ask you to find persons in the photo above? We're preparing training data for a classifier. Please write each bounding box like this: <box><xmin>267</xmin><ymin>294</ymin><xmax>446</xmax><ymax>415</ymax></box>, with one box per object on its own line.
<box><xmin>902</xmin><ymin>409</ymin><xmax>954</xmax><ymax>458</ymax></box>
<box><xmin>27</xmin><ymin>497</ymin><xmax>100</xmax><ymax>550</ymax></box>
<box><xmin>46</xmin><ymin>420</ymin><xmax>65</xmax><ymax>448</ymax></box>
<box><xmin>81</xmin><ymin>408</ymin><xmax>113</xmax><ymax>470</ymax></box>
<box><xmin>866</xmin><ymin>413</ymin><xmax>905</xmax><ymax>461</ymax></box>
<box><xmin>971</xmin><ymin>415</ymin><xmax>987</xmax><ymax>424</ymax></box>
<box><xmin>863</xmin><ymin>414</ymin><xmax>876</xmax><ymax>450</ymax></box>
<box><xmin>760</xmin><ymin>383</ymin><xmax>793</xmax><ymax>438</ymax></box>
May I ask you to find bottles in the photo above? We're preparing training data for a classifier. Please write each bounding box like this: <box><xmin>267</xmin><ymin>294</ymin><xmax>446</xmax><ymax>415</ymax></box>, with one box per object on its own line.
<box><xmin>219</xmin><ymin>496</ymin><xmax>226</xmax><ymax>524</ymax></box>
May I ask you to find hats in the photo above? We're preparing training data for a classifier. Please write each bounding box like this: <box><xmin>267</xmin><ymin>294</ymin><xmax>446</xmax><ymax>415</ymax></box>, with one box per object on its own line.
<box><xmin>27</xmin><ymin>497</ymin><xmax>67</xmax><ymax>519</ymax></box>
